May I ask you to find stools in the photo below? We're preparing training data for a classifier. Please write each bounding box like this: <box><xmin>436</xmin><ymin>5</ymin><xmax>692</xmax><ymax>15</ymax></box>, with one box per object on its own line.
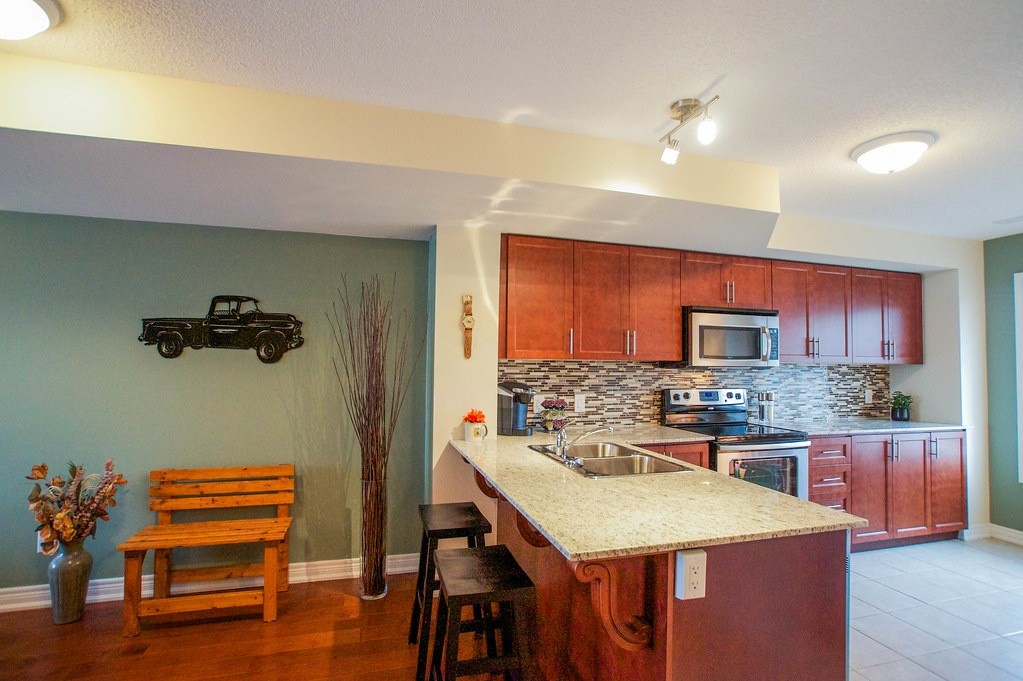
<box><xmin>408</xmin><ymin>502</ymin><xmax>538</xmax><ymax>681</ymax></box>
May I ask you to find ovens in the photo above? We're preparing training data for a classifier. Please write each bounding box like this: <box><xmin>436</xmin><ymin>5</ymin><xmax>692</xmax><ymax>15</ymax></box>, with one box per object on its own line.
<box><xmin>709</xmin><ymin>441</ymin><xmax>811</xmax><ymax>501</ymax></box>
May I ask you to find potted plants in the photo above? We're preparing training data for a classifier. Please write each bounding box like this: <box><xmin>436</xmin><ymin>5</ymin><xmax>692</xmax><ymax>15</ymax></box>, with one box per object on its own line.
<box><xmin>885</xmin><ymin>390</ymin><xmax>913</xmax><ymax>421</ymax></box>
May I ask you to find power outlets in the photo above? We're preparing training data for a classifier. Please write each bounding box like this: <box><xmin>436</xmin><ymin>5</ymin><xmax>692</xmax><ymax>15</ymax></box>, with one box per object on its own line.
<box><xmin>675</xmin><ymin>549</ymin><xmax>707</xmax><ymax>600</ymax></box>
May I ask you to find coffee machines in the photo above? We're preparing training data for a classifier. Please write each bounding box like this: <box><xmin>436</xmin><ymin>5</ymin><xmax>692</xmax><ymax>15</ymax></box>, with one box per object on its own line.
<box><xmin>498</xmin><ymin>380</ymin><xmax>532</xmax><ymax>436</ymax></box>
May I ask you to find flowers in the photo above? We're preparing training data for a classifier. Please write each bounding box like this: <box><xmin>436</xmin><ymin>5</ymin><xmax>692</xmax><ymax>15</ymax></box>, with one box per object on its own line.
<box><xmin>463</xmin><ymin>409</ymin><xmax>485</xmax><ymax>424</ymax></box>
<box><xmin>25</xmin><ymin>458</ymin><xmax>128</xmax><ymax>555</ymax></box>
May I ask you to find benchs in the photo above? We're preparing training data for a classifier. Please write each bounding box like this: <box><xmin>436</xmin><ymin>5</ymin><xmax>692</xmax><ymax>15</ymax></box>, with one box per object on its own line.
<box><xmin>116</xmin><ymin>464</ymin><xmax>295</xmax><ymax>637</ymax></box>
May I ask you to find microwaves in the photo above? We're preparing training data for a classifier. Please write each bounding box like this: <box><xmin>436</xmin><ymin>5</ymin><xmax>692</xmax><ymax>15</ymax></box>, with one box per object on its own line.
<box><xmin>659</xmin><ymin>306</ymin><xmax>780</xmax><ymax>369</ymax></box>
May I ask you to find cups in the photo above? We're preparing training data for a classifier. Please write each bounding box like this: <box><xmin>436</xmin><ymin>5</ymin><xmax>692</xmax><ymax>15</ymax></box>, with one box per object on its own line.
<box><xmin>464</xmin><ymin>422</ymin><xmax>488</xmax><ymax>441</ymax></box>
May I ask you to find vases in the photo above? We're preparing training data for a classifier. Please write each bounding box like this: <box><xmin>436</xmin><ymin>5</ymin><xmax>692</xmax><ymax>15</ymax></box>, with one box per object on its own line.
<box><xmin>464</xmin><ymin>422</ymin><xmax>488</xmax><ymax>442</ymax></box>
<box><xmin>48</xmin><ymin>538</ymin><xmax>97</xmax><ymax>624</ymax></box>
<box><xmin>358</xmin><ymin>477</ymin><xmax>390</xmax><ymax>601</ymax></box>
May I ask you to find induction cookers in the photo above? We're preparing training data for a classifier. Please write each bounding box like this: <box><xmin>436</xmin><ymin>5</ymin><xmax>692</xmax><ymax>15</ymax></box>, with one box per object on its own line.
<box><xmin>662</xmin><ymin>389</ymin><xmax>809</xmax><ymax>442</ymax></box>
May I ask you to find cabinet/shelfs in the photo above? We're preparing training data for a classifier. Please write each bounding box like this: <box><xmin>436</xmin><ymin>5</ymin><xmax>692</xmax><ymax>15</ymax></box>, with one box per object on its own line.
<box><xmin>499</xmin><ymin>233</ymin><xmax>925</xmax><ymax>366</ymax></box>
<box><xmin>808</xmin><ymin>436</ymin><xmax>852</xmax><ymax>514</ymax></box>
<box><xmin>852</xmin><ymin>430</ymin><xmax>968</xmax><ymax>553</ymax></box>
<box><xmin>640</xmin><ymin>442</ymin><xmax>710</xmax><ymax>469</ymax></box>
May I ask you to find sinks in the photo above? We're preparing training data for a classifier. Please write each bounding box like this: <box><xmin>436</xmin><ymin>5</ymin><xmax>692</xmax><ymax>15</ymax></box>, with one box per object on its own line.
<box><xmin>527</xmin><ymin>438</ymin><xmax>660</xmax><ymax>457</ymax></box>
<box><xmin>566</xmin><ymin>461</ymin><xmax>699</xmax><ymax>480</ymax></box>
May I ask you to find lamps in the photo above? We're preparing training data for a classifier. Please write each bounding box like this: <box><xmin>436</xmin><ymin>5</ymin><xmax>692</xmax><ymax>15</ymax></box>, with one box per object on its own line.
<box><xmin>0</xmin><ymin>0</ymin><xmax>63</xmax><ymax>42</ymax></box>
<box><xmin>851</xmin><ymin>132</ymin><xmax>935</xmax><ymax>174</ymax></box>
<box><xmin>658</xmin><ymin>95</ymin><xmax>720</xmax><ymax>165</ymax></box>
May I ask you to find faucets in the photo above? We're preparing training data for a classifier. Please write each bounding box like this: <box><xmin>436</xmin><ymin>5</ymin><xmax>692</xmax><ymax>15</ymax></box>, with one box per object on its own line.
<box><xmin>555</xmin><ymin>419</ymin><xmax>615</xmax><ymax>459</ymax></box>
